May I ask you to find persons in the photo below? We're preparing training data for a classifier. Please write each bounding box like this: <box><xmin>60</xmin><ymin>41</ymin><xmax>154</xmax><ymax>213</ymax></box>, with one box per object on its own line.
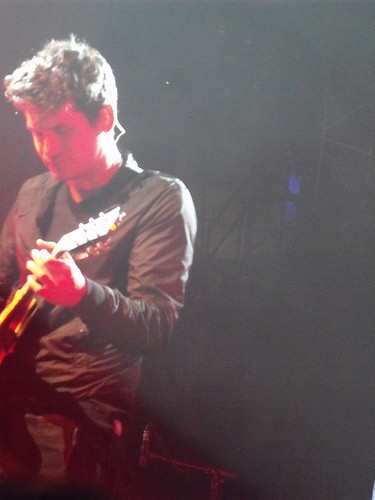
<box><xmin>0</xmin><ymin>35</ymin><xmax>197</xmax><ymax>500</ymax></box>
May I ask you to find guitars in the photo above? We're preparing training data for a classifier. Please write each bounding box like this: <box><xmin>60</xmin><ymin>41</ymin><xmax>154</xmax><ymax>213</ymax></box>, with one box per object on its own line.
<box><xmin>0</xmin><ymin>206</ymin><xmax>126</xmax><ymax>364</ymax></box>
